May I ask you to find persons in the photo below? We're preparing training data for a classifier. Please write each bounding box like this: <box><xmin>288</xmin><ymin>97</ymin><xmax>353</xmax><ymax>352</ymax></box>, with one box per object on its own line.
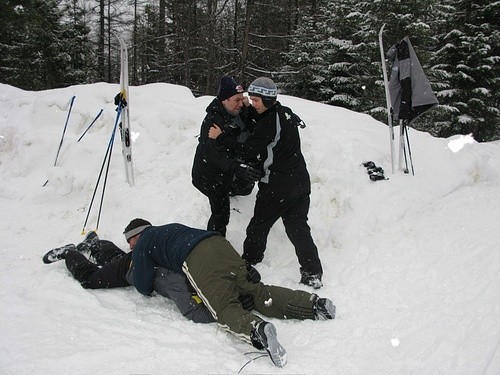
<box><xmin>43</xmin><ymin>231</ymin><xmax>259</xmax><ymax>324</ymax></box>
<box><xmin>123</xmin><ymin>218</ymin><xmax>336</xmax><ymax>368</ymax></box>
<box><xmin>208</xmin><ymin>78</ymin><xmax>324</xmax><ymax>290</ymax></box>
<box><xmin>190</xmin><ymin>76</ymin><xmax>306</xmax><ymax>236</ymax></box>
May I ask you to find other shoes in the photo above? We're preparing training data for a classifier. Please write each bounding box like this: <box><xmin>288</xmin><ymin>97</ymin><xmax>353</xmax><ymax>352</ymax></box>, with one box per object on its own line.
<box><xmin>299</xmin><ymin>268</ymin><xmax>323</xmax><ymax>289</ymax></box>
<box><xmin>316</xmin><ymin>298</ymin><xmax>336</xmax><ymax>319</ymax></box>
<box><xmin>238</xmin><ymin>321</ymin><xmax>287</xmax><ymax>374</ymax></box>
<box><xmin>243</xmin><ymin>254</ymin><xmax>264</xmax><ymax>266</ymax></box>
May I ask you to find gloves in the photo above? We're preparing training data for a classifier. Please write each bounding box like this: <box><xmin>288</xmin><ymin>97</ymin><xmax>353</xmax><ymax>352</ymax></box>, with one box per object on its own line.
<box><xmin>246</xmin><ymin>265</ymin><xmax>261</xmax><ymax>284</ymax></box>
<box><xmin>240</xmin><ymin>294</ymin><xmax>255</xmax><ymax>311</ymax></box>
<box><xmin>235</xmin><ymin>165</ymin><xmax>265</xmax><ymax>183</ymax></box>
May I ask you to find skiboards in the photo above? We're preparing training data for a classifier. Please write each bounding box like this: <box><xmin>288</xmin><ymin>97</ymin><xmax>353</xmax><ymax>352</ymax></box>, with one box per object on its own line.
<box><xmin>378</xmin><ymin>24</ymin><xmax>404</xmax><ymax>176</ymax></box>
<box><xmin>115</xmin><ymin>32</ymin><xmax>137</xmax><ymax>188</ymax></box>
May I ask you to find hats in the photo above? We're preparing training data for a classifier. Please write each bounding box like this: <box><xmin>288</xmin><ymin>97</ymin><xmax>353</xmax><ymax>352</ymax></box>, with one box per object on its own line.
<box><xmin>248</xmin><ymin>77</ymin><xmax>277</xmax><ymax>98</ymax></box>
<box><xmin>218</xmin><ymin>74</ymin><xmax>244</xmax><ymax>101</ymax></box>
<box><xmin>123</xmin><ymin>218</ymin><xmax>152</xmax><ymax>242</ymax></box>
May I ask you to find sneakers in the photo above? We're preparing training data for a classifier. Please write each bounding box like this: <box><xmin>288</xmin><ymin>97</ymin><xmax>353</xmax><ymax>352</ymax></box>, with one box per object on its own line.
<box><xmin>76</xmin><ymin>231</ymin><xmax>99</xmax><ymax>250</ymax></box>
<box><xmin>43</xmin><ymin>244</ymin><xmax>75</xmax><ymax>264</ymax></box>
